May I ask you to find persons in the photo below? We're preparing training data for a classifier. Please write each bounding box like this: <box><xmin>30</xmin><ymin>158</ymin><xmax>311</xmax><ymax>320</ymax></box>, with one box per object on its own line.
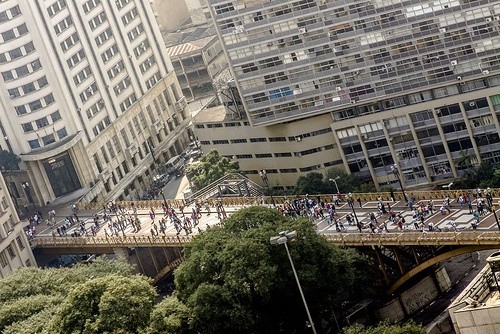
<box><xmin>23</xmin><ymin>183</ymin><xmax>500</xmax><ymax>252</ymax></box>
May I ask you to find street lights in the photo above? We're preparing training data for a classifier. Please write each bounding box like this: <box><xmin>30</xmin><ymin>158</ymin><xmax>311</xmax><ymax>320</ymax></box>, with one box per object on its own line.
<box><xmin>484</xmin><ymin>187</ymin><xmax>500</xmax><ymax>231</ymax></box>
<box><xmin>257</xmin><ymin>169</ymin><xmax>277</xmax><ymax>208</ymax></box>
<box><xmin>329</xmin><ymin>176</ymin><xmax>341</xmax><ymax>194</ymax></box>
<box><xmin>390</xmin><ymin>164</ymin><xmax>408</xmax><ymax>202</ymax></box>
<box><xmin>344</xmin><ymin>192</ymin><xmax>362</xmax><ymax>233</ymax></box>
<box><xmin>269</xmin><ymin>230</ymin><xmax>318</xmax><ymax>334</ymax></box>
<box><xmin>152</xmin><ymin>174</ymin><xmax>169</xmax><ymax>209</ymax></box>
<box><xmin>442</xmin><ymin>183</ymin><xmax>453</xmax><ymax>190</ymax></box>
<box><xmin>23</xmin><ymin>182</ymin><xmax>37</xmax><ymax>209</ymax></box>
<box><xmin>109</xmin><ymin>199</ymin><xmax>125</xmax><ymax>236</ymax></box>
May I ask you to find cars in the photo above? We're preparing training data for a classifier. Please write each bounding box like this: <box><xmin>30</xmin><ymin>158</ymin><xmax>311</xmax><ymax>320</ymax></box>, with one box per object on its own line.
<box><xmin>174</xmin><ymin>164</ymin><xmax>187</xmax><ymax>178</ymax></box>
<box><xmin>180</xmin><ymin>140</ymin><xmax>202</xmax><ymax>159</ymax></box>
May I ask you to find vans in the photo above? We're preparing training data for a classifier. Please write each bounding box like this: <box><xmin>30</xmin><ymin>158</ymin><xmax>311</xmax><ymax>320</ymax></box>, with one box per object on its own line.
<box><xmin>164</xmin><ymin>155</ymin><xmax>186</xmax><ymax>173</ymax></box>
<box><xmin>156</xmin><ymin>173</ymin><xmax>170</xmax><ymax>186</ymax></box>
